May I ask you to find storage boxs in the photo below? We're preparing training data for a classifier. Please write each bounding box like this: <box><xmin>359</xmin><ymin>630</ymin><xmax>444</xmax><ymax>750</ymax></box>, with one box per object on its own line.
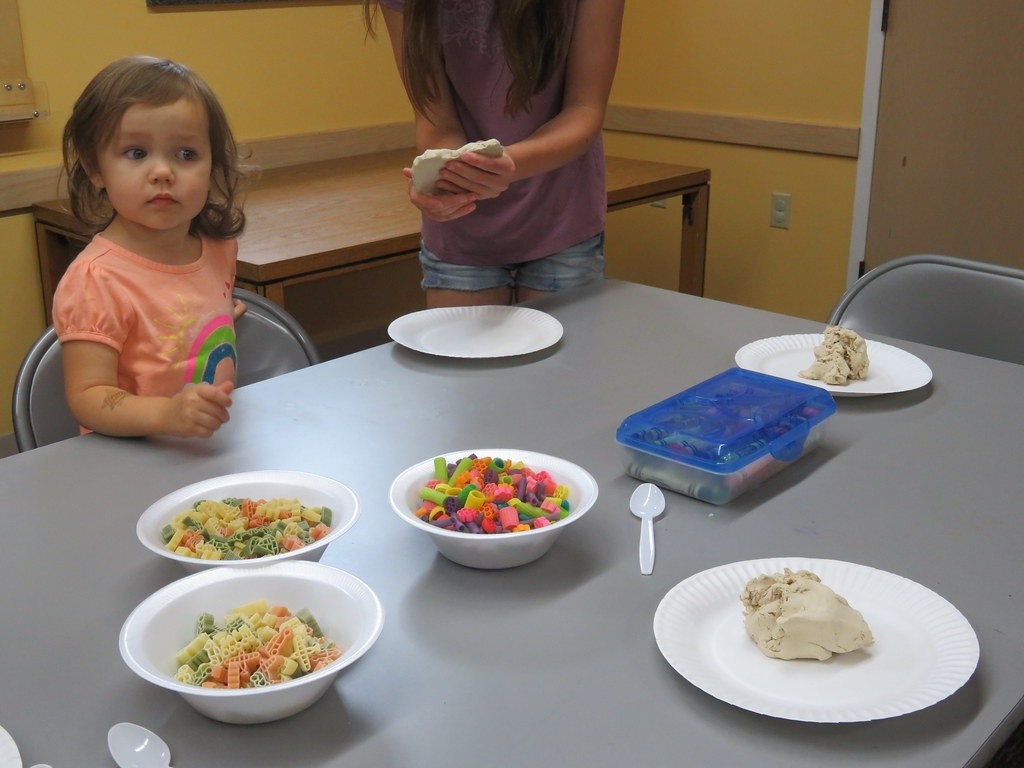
<box><xmin>611</xmin><ymin>368</ymin><xmax>836</xmax><ymax>508</ymax></box>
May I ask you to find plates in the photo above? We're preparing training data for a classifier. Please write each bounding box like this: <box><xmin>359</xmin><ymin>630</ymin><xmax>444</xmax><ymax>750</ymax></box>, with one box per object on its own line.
<box><xmin>387</xmin><ymin>306</ymin><xmax>563</xmax><ymax>359</ymax></box>
<box><xmin>652</xmin><ymin>556</ymin><xmax>980</xmax><ymax>723</ymax></box>
<box><xmin>735</xmin><ymin>334</ymin><xmax>932</xmax><ymax>397</ymax></box>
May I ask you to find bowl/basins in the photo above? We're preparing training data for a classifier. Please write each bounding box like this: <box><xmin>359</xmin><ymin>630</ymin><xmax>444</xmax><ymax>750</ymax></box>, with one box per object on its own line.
<box><xmin>389</xmin><ymin>448</ymin><xmax>599</xmax><ymax>569</ymax></box>
<box><xmin>119</xmin><ymin>560</ymin><xmax>385</xmax><ymax>724</ymax></box>
<box><xmin>135</xmin><ymin>471</ymin><xmax>361</xmax><ymax>573</ymax></box>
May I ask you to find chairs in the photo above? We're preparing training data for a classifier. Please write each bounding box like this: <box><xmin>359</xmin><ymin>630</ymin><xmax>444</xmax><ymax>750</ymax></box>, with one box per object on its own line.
<box><xmin>11</xmin><ymin>287</ymin><xmax>322</xmax><ymax>455</ymax></box>
<box><xmin>826</xmin><ymin>253</ymin><xmax>1024</xmax><ymax>364</ymax></box>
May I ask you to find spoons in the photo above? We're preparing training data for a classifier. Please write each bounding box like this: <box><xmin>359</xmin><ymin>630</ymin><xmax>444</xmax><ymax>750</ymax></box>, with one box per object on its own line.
<box><xmin>107</xmin><ymin>722</ymin><xmax>174</xmax><ymax>768</ymax></box>
<box><xmin>629</xmin><ymin>483</ymin><xmax>665</xmax><ymax>575</ymax></box>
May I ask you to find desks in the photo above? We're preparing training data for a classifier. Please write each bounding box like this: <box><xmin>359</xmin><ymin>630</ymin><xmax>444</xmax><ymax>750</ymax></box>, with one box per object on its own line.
<box><xmin>0</xmin><ymin>275</ymin><xmax>1024</xmax><ymax>768</ymax></box>
<box><xmin>30</xmin><ymin>146</ymin><xmax>712</xmax><ymax>329</ymax></box>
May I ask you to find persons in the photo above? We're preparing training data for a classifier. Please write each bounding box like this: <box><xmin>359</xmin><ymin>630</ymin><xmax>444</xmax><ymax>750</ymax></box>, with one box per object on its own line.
<box><xmin>362</xmin><ymin>1</ymin><xmax>621</xmax><ymax>319</ymax></box>
<box><xmin>52</xmin><ymin>54</ymin><xmax>248</xmax><ymax>436</ymax></box>
